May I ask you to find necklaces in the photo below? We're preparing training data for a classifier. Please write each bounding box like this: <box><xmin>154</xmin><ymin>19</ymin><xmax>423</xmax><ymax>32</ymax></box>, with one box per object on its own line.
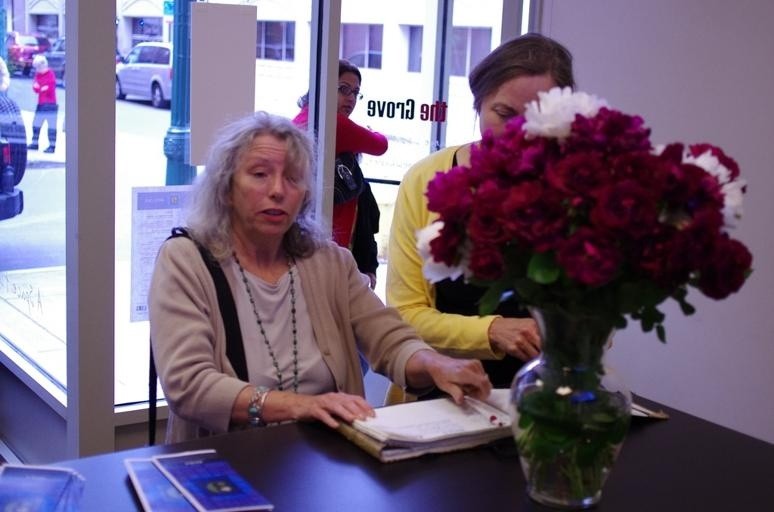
<box><xmin>226</xmin><ymin>233</ymin><xmax>298</xmax><ymax>418</ymax></box>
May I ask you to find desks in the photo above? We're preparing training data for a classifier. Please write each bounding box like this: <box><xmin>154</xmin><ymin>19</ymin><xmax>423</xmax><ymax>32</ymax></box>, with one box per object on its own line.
<box><xmin>0</xmin><ymin>381</ymin><xmax>774</xmax><ymax>512</ymax></box>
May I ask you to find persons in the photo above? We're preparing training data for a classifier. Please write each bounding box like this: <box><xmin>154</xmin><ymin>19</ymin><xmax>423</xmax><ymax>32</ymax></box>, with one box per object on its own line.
<box><xmin>385</xmin><ymin>34</ymin><xmax>576</xmax><ymax>409</ymax></box>
<box><xmin>289</xmin><ymin>59</ymin><xmax>389</xmax><ymax>250</ymax></box>
<box><xmin>351</xmin><ymin>151</ymin><xmax>380</xmax><ymax>377</ymax></box>
<box><xmin>148</xmin><ymin>110</ymin><xmax>493</xmax><ymax>446</ymax></box>
<box><xmin>27</xmin><ymin>55</ymin><xmax>58</xmax><ymax>153</ymax></box>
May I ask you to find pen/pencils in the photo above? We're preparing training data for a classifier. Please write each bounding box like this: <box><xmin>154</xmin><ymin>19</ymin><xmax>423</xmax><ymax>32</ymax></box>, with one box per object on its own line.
<box><xmin>465</xmin><ymin>399</ymin><xmax>503</xmax><ymax>430</ymax></box>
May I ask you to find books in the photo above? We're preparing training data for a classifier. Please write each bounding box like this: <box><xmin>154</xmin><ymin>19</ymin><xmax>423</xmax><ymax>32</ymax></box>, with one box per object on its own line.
<box><xmin>353</xmin><ymin>388</ymin><xmax>665</xmax><ymax>445</ymax></box>
<box><xmin>336</xmin><ymin>418</ymin><xmax>514</xmax><ymax>460</ymax></box>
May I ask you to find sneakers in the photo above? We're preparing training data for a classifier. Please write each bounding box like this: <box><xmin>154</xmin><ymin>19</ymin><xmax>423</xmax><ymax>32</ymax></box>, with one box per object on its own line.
<box><xmin>44</xmin><ymin>145</ymin><xmax>55</xmax><ymax>153</ymax></box>
<box><xmin>27</xmin><ymin>143</ymin><xmax>37</xmax><ymax>148</ymax></box>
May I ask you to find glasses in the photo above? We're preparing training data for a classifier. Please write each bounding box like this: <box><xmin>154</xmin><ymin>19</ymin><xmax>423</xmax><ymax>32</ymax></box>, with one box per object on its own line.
<box><xmin>337</xmin><ymin>84</ymin><xmax>363</xmax><ymax>101</ymax></box>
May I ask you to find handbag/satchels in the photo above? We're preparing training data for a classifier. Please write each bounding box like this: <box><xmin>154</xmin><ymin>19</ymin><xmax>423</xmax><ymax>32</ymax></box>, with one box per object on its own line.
<box><xmin>333</xmin><ymin>151</ymin><xmax>368</xmax><ymax>207</ymax></box>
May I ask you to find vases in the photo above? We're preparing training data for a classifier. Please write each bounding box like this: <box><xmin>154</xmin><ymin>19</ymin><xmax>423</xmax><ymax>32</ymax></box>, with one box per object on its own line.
<box><xmin>509</xmin><ymin>303</ymin><xmax>632</xmax><ymax>512</ymax></box>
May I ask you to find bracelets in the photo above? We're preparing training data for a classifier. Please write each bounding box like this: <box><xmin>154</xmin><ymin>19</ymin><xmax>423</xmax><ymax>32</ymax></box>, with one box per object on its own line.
<box><xmin>248</xmin><ymin>383</ymin><xmax>273</xmax><ymax>428</ymax></box>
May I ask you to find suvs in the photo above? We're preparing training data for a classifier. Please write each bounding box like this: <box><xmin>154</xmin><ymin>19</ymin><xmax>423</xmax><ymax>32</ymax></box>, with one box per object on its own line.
<box><xmin>6</xmin><ymin>31</ymin><xmax>51</xmax><ymax>75</ymax></box>
<box><xmin>0</xmin><ymin>93</ymin><xmax>28</xmax><ymax>219</ymax></box>
<box><xmin>115</xmin><ymin>42</ymin><xmax>173</xmax><ymax>108</ymax></box>
<box><xmin>30</xmin><ymin>36</ymin><xmax>66</xmax><ymax>88</ymax></box>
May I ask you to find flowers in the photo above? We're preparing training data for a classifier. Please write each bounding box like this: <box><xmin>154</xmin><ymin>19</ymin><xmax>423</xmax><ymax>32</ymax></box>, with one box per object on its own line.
<box><xmin>412</xmin><ymin>87</ymin><xmax>753</xmax><ymax>348</ymax></box>
